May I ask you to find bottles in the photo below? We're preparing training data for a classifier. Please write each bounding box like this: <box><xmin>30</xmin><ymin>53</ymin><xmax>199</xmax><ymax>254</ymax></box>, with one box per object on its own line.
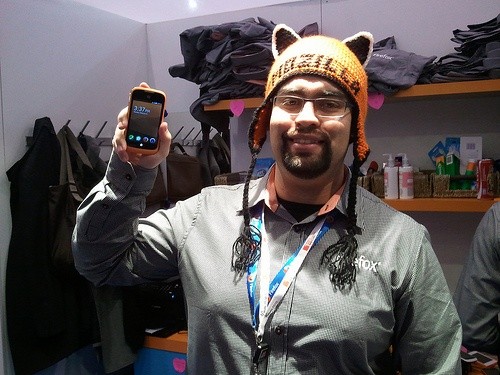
<box><xmin>383</xmin><ymin>154</ymin><xmax>416</xmax><ymax>200</ymax></box>
<box><xmin>367</xmin><ymin>160</ymin><xmax>379</xmax><ymax>174</ymax></box>
<box><xmin>460</xmin><ymin>158</ymin><xmax>477</xmax><ymax>190</ymax></box>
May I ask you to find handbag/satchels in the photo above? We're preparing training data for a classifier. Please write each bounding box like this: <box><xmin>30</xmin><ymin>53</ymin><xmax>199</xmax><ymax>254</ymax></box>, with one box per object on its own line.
<box><xmin>166</xmin><ymin>142</ymin><xmax>202</xmax><ymax>202</ymax></box>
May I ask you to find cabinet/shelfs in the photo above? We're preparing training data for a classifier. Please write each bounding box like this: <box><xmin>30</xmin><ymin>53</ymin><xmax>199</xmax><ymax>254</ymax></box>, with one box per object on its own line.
<box><xmin>204</xmin><ymin>77</ymin><xmax>500</xmax><ymax>212</ymax></box>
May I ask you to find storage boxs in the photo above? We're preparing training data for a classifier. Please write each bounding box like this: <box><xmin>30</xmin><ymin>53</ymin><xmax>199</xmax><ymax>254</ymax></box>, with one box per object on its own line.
<box><xmin>478</xmin><ymin>158</ymin><xmax>494</xmax><ymax>199</ymax></box>
<box><xmin>370</xmin><ymin>173</ymin><xmax>384</xmax><ymax>198</ymax></box>
<box><xmin>432</xmin><ymin>172</ymin><xmax>479</xmax><ymax>198</ymax></box>
<box><xmin>427</xmin><ymin>140</ymin><xmax>446</xmax><ymax>168</ymax></box>
<box><xmin>459</xmin><ymin>135</ymin><xmax>483</xmax><ymax>176</ymax></box>
<box><xmin>357</xmin><ymin>176</ymin><xmax>362</xmax><ymax>187</ymax></box>
<box><xmin>445</xmin><ymin>154</ymin><xmax>460</xmax><ymax>176</ymax></box>
<box><xmin>251</xmin><ymin>157</ymin><xmax>274</xmax><ymax>176</ymax></box>
<box><xmin>436</xmin><ymin>155</ymin><xmax>446</xmax><ymax>175</ymax></box>
<box><xmin>363</xmin><ymin>176</ymin><xmax>369</xmax><ymax>191</ymax></box>
<box><xmin>412</xmin><ymin>172</ymin><xmax>434</xmax><ymax>198</ymax></box>
<box><xmin>214</xmin><ymin>170</ymin><xmax>246</xmax><ymax>185</ymax></box>
<box><xmin>445</xmin><ymin>136</ymin><xmax>460</xmax><ymax>158</ymax></box>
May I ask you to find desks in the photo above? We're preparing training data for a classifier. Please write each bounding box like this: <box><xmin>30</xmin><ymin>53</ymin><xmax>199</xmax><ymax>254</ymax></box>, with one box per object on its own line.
<box><xmin>133</xmin><ymin>329</ymin><xmax>188</xmax><ymax>375</ymax></box>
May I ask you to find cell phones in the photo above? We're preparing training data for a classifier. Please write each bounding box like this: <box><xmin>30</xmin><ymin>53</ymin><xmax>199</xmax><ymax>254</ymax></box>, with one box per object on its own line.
<box><xmin>124</xmin><ymin>87</ymin><xmax>167</xmax><ymax>155</ymax></box>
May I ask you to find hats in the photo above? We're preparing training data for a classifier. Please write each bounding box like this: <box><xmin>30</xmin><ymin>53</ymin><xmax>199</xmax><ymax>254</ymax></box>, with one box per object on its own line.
<box><xmin>231</xmin><ymin>24</ymin><xmax>374</xmax><ymax>291</ymax></box>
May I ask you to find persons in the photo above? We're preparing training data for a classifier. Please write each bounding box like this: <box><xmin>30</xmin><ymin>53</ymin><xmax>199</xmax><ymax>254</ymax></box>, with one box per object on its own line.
<box><xmin>71</xmin><ymin>24</ymin><xmax>462</xmax><ymax>375</ymax></box>
<box><xmin>452</xmin><ymin>202</ymin><xmax>500</xmax><ymax>357</ymax></box>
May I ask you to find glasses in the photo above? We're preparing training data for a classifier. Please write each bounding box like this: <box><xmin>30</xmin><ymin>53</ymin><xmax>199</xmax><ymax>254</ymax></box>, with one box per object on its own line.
<box><xmin>270</xmin><ymin>95</ymin><xmax>352</xmax><ymax>118</ymax></box>
